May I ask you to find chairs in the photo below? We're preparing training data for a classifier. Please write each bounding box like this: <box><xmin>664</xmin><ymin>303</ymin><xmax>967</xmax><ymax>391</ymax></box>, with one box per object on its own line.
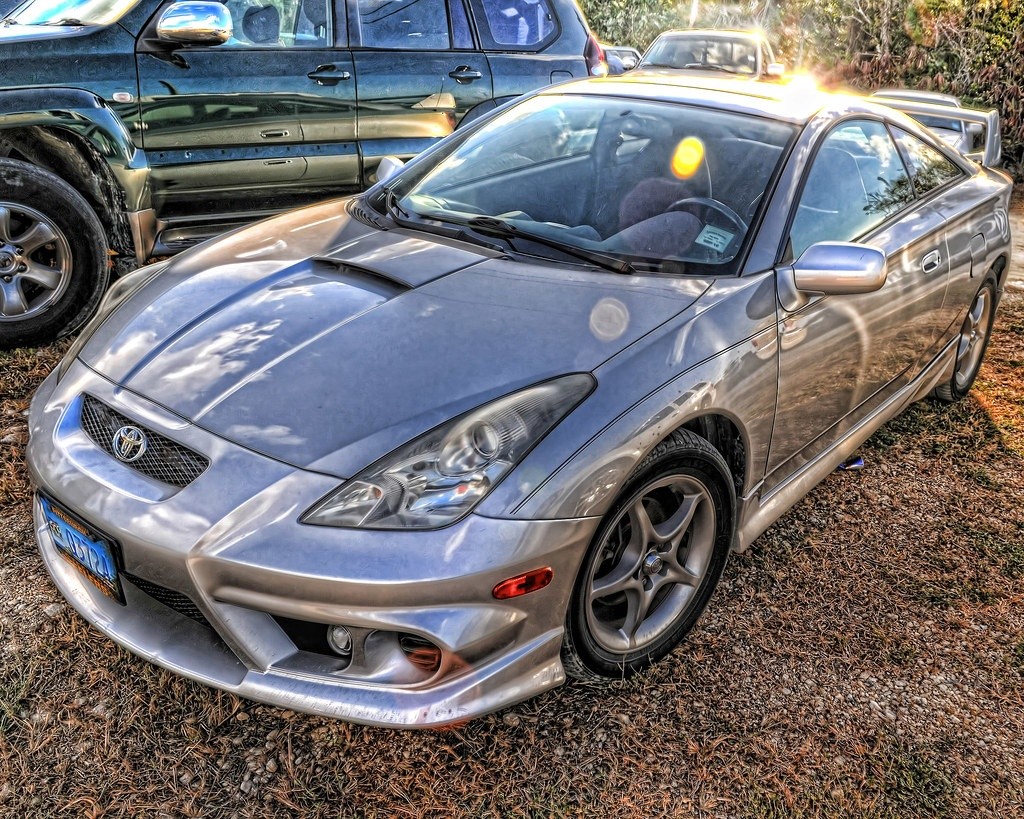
<box><xmin>289</xmin><ymin>0</ymin><xmax>326</xmax><ymax>44</ymax></box>
<box><xmin>746</xmin><ymin>148</ymin><xmax>870</xmax><ymax>263</ymax></box>
<box><xmin>585</xmin><ymin>133</ymin><xmax>713</xmax><ymax>251</ymax></box>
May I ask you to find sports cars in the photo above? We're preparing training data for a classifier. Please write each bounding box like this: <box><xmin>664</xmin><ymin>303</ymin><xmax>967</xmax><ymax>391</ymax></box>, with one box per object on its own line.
<box><xmin>26</xmin><ymin>77</ymin><xmax>1013</xmax><ymax>733</ymax></box>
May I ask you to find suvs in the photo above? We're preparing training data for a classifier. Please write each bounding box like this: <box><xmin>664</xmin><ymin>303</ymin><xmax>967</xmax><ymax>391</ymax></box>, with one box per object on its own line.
<box><xmin>0</xmin><ymin>0</ymin><xmax>620</xmax><ymax>354</ymax></box>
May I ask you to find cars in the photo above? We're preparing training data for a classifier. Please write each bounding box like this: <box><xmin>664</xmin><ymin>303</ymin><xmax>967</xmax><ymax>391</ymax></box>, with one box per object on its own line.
<box><xmin>633</xmin><ymin>24</ymin><xmax>783</xmax><ymax>84</ymax></box>
<box><xmin>600</xmin><ymin>43</ymin><xmax>642</xmax><ymax>71</ymax></box>
<box><xmin>870</xmin><ymin>85</ymin><xmax>1002</xmax><ymax>176</ymax></box>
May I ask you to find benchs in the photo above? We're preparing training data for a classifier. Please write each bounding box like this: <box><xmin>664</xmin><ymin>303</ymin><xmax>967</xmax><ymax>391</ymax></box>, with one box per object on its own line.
<box><xmin>678</xmin><ymin>119</ymin><xmax>881</xmax><ymax>235</ymax></box>
